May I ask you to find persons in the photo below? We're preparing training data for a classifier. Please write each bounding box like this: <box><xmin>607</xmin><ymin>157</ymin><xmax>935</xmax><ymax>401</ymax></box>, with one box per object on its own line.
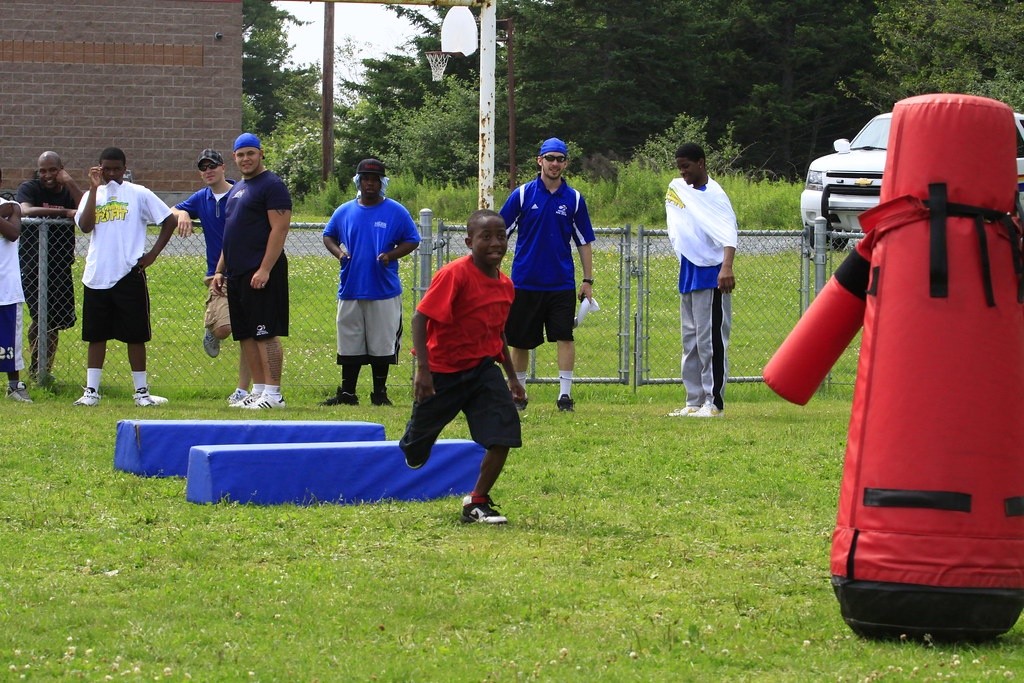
<box><xmin>500</xmin><ymin>137</ymin><xmax>596</xmax><ymax>411</ymax></box>
<box><xmin>665</xmin><ymin>142</ymin><xmax>737</xmax><ymax>417</ymax></box>
<box><xmin>74</xmin><ymin>147</ymin><xmax>178</xmax><ymax>405</ymax></box>
<box><xmin>318</xmin><ymin>159</ymin><xmax>421</xmax><ymax>405</ymax></box>
<box><xmin>400</xmin><ymin>209</ymin><xmax>525</xmax><ymax>524</ymax></box>
<box><xmin>212</xmin><ymin>133</ymin><xmax>292</xmax><ymax>409</ymax></box>
<box><xmin>170</xmin><ymin>149</ymin><xmax>251</xmax><ymax>404</ymax></box>
<box><xmin>16</xmin><ymin>152</ymin><xmax>83</xmax><ymax>382</ymax></box>
<box><xmin>0</xmin><ymin>168</ymin><xmax>32</xmax><ymax>402</ymax></box>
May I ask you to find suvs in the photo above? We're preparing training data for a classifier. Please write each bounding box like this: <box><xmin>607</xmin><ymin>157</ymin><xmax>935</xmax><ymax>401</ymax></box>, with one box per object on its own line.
<box><xmin>800</xmin><ymin>112</ymin><xmax>1024</xmax><ymax>252</ymax></box>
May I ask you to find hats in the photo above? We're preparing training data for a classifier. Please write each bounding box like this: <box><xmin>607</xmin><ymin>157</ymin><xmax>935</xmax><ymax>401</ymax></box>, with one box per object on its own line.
<box><xmin>234</xmin><ymin>133</ymin><xmax>265</xmax><ymax>160</ymax></box>
<box><xmin>198</xmin><ymin>148</ymin><xmax>224</xmax><ymax>166</ymax></box>
<box><xmin>540</xmin><ymin>138</ymin><xmax>567</xmax><ymax>156</ymax></box>
<box><xmin>357</xmin><ymin>160</ymin><xmax>386</xmax><ymax>177</ymax></box>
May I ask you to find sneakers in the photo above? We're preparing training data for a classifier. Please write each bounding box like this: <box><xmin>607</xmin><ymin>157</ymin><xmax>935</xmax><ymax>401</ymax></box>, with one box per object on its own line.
<box><xmin>225</xmin><ymin>390</ymin><xmax>242</xmax><ymax>403</ymax></box>
<box><xmin>243</xmin><ymin>396</ymin><xmax>284</xmax><ymax>409</ymax></box>
<box><xmin>405</xmin><ymin>448</ymin><xmax>432</xmax><ymax>469</ymax></box>
<box><xmin>669</xmin><ymin>402</ymin><xmax>701</xmax><ymax>417</ymax></box>
<box><xmin>73</xmin><ymin>387</ymin><xmax>102</xmax><ymax>406</ymax></box>
<box><xmin>688</xmin><ymin>403</ymin><xmax>723</xmax><ymax>418</ymax></box>
<box><xmin>514</xmin><ymin>395</ymin><xmax>529</xmax><ymax>411</ymax></box>
<box><xmin>556</xmin><ymin>392</ymin><xmax>575</xmax><ymax>413</ymax></box>
<box><xmin>369</xmin><ymin>391</ymin><xmax>391</xmax><ymax>405</ymax></box>
<box><xmin>131</xmin><ymin>387</ymin><xmax>157</xmax><ymax>406</ymax></box>
<box><xmin>5</xmin><ymin>383</ymin><xmax>31</xmax><ymax>403</ymax></box>
<box><xmin>321</xmin><ymin>388</ymin><xmax>360</xmax><ymax>406</ymax></box>
<box><xmin>462</xmin><ymin>495</ymin><xmax>507</xmax><ymax>525</ymax></box>
<box><xmin>228</xmin><ymin>391</ymin><xmax>261</xmax><ymax>406</ymax></box>
<box><xmin>203</xmin><ymin>324</ymin><xmax>220</xmax><ymax>359</ymax></box>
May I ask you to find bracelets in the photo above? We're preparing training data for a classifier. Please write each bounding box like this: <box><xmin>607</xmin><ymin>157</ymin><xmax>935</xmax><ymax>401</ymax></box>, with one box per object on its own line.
<box><xmin>215</xmin><ymin>271</ymin><xmax>224</xmax><ymax>275</ymax></box>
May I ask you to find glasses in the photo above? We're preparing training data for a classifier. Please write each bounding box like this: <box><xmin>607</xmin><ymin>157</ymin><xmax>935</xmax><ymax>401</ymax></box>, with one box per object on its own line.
<box><xmin>200</xmin><ymin>163</ymin><xmax>219</xmax><ymax>171</ymax></box>
<box><xmin>543</xmin><ymin>154</ymin><xmax>568</xmax><ymax>162</ymax></box>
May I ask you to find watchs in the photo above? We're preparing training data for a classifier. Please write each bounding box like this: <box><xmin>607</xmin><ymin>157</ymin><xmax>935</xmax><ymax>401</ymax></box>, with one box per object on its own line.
<box><xmin>583</xmin><ymin>279</ymin><xmax>594</xmax><ymax>285</ymax></box>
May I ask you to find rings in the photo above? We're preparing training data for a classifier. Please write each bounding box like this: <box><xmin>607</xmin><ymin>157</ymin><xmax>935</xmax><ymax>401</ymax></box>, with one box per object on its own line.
<box><xmin>262</xmin><ymin>284</ymin><xmax>264</xmax><ymax>286</ymax></box>
